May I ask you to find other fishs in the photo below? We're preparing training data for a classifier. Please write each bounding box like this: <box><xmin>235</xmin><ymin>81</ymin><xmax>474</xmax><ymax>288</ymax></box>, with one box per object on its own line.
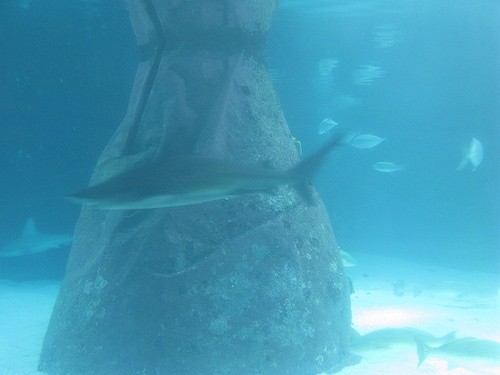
<box><xmin>415</xmin><ymin>335</ymin><xmax>500</xmax><ymax>367</ymax></box>
<box><xmin>372</xmin><ymin>162</ymin><xmax>401</xmax><ymax>173</ymax></box>
<box><xmin>64</xmin><ymin>50</ymin><xmax>343</xmax><ymax>210</ymax></box>
<box><xmin>351</xmin><ymin>134</ymin><xmax>385</xmax><ymax>150</ymax></box>
<box><xmin>0</xmin><ymin>217</ymin><xmax>73</xmax><ymax>258</ymax></box>
<box><xmin>353</xmin><ymin>327</ymin><xmax>475</xmax><ymax>351</ymax></box>
<box><xmin>457</xmin><ymin>137</ymin><xmax>483</xmax><ymax>173</ymax></box>
<box><xmin>317</xmin><ymin>118</ymin><xmax>338</xmax><ymax>134</ymax></box>
<box><xmin>338</xmin><ymin>249</ymin><xmax>357</xmax><ymax>268</ymax></box>
